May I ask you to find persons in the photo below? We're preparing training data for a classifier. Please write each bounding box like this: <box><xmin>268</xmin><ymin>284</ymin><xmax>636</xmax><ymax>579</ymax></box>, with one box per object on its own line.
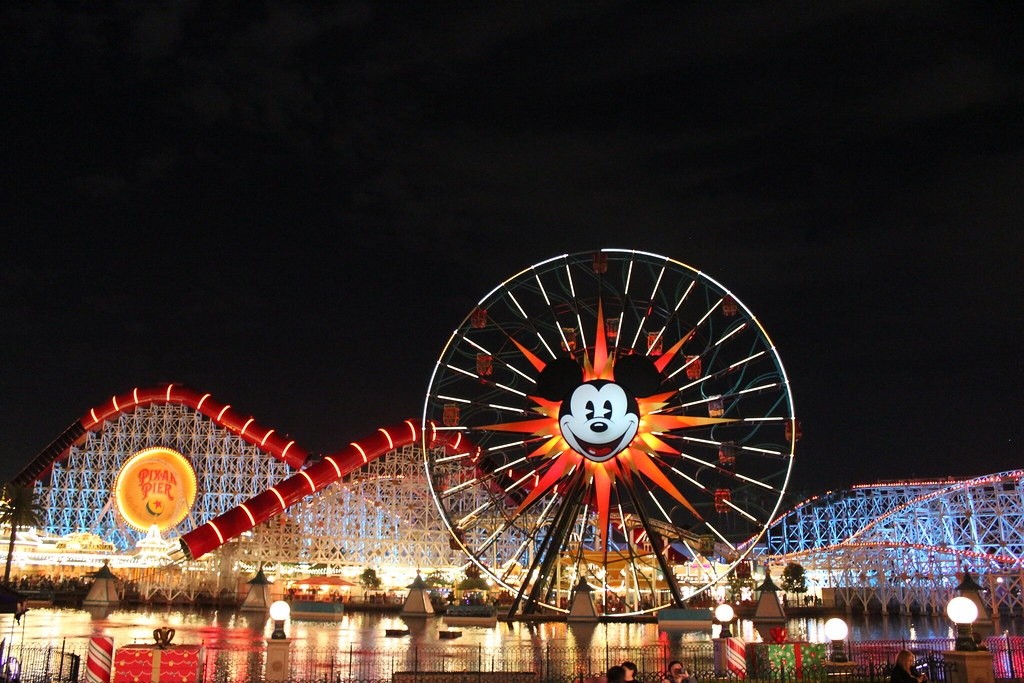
<box><xmin>662</xmin><ymin>661</ymin><xmax>697</xmax><ymax>683</ymax></box>
<box><xmin>804</xmin><ymin>594</ymin><xmax>818</xmax><ymax>607</ymax></box>
<box><xmin>14</xmin><ymin>574</ymin><xmax>84</xmax><ymax>591</ymax></box>
<box><xmin>890</xmin><ymin>650</ymin><xmax>928</xmax><ymax>683</ymax></box>
<box><xmin>783</xmin><ymin>594</ymin><xmax>786</xmax><ymax>602</ymax></box>
<box><xmin>348</xmin><ymin>592</ymin><xmax>352</xmax><ymax>602</ymax></box>
<box><xmin>364</xmin><ymin>591</ymin><xmax>367</xmax><ymax>599</ymax></box>
<box><xmin>312</xmin><ymin>587</ymin><xmax>316</xmax><ymax>594</ymax></box>
<box><xmin>375</xmin><ymin>592</ymin><xmax>386</xmax><ymax>604</ymax></box>
<box><xmin>619</xmin><ymin>594</ymin><xmax>626</xmax><ymax>601</ymax></box>
<box><xmin>332</xmin><ymin>591</ymin><xmax>339</xmax><ymax>602</ymax></box>
<box><xmin>607</xmin><ymin>662</ymin><xmax>642</xmax><ymax>683</ymax></box>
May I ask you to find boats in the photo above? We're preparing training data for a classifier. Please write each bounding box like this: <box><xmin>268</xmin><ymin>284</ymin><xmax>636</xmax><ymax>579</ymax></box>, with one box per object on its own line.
<box><xmin>15</xmin><ymin>590</ymin><xmax>54</xmax><ymax>607</ymax></box>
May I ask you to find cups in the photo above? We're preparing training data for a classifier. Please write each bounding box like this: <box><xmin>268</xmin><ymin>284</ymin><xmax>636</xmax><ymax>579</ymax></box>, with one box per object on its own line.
<box><xmin>678</xmin><ymin>668</ymin><xmax>685</xmax><ymax>674</ymax></box>
<box><xmin>921</xmin><ymin>673</ymin><xmax>925</xmax><ymax>680</ymax></box>
